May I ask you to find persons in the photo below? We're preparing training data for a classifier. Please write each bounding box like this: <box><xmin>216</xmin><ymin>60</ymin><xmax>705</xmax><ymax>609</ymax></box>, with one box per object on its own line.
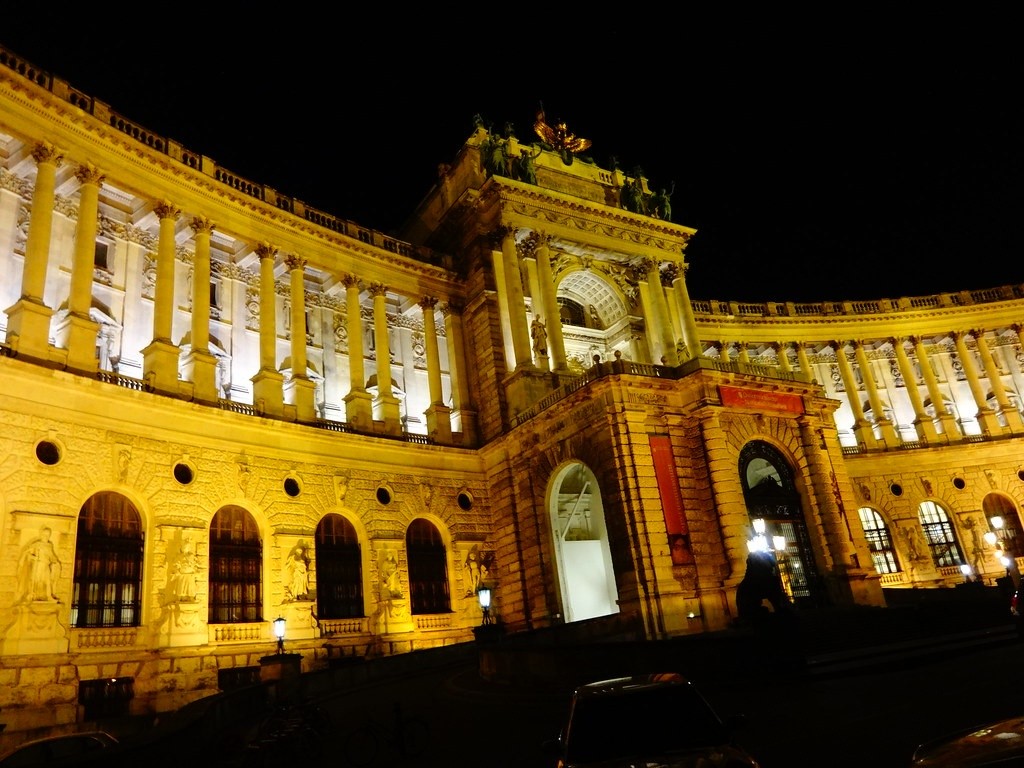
<box><xmin>171</xmin><ymin>538</ymin><xmax>198</xmax><ymax>602</ymax></box>
<box><xmin>677</xmin><ymin>338</ymin><xmax>690</xmax><ymax>364</ymax></box>
<box><xmin>489</xmin><ymin>127</ymin><xmax>509</xmax><ymax>177</ymax></box>
<box><xmin>285</xmin><ymin>547</ymin><xmax>310</xmax><ymax>602</ymax></box>
<box><xmin>971</xmin><ymin>520</ymin><xmax>986</xmax><ymax>549</ymax></box>
<box><xmin>382</xmin><ymin>552</ymin><xmax>404</xmax><ymax>598</ymax></box>
<box><xmin>516</xmin><ymin>143</ymin><xmax>542</xmax><ymax>185</ymax></box>
<box><xmin>464</xmin><ymin>553</ymin><xmax>481</xmax><ymax>596</ymax></box>
<box><xmin>659</xmin><ymin>182</ymin><xmax>675</xmax><ymax>221</ymax></box>
<box><xmin>590</xmin><ymin>309</ymin><xmax>601</xmax><ymax>329</ymax></box>
<box><xmin>905</xmin><ymin>526</ymin><xmax>923</xmax><ymax>557</ymax></box>
<box><xmin>24</xmin><ymin>528</ymin><xmax>61</xmax><ymax>604</ymax></box>
<box><xmin>531</xmin><ymin>315</ymin><xmax>548</xmax><ymax>356</ymax></box>
<box><xmin>629</xmin><ymin>179</ymin><xmax>646</xmax><ymax>214</ymax></box>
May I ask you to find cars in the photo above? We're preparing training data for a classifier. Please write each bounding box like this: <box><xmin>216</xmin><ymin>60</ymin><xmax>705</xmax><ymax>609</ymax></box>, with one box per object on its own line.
<box><xmin>539</xmin><ymin>672</ymin><xmax>764</xmax><ymax>768</ymax></box>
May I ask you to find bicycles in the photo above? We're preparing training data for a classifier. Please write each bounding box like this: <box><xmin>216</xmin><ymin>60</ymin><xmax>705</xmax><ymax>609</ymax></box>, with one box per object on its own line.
<box><xmin>342</xmin><ymin>702</ymin><xmax>433</xmax><ymax>768</ymax></box>
<box><xmin>207</xmin><ymin>692</ymin><xmax>339</xmax><ymax>768</ymax></box>
